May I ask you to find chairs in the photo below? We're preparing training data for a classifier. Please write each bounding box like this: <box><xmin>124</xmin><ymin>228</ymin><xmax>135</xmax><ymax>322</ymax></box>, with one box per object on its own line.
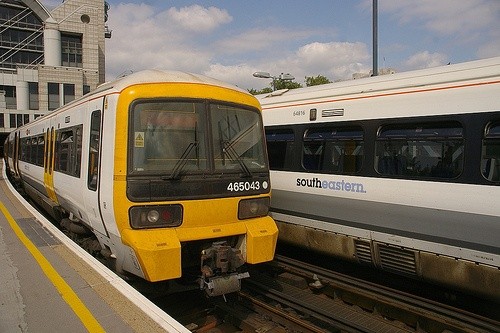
<box><xmin>304</xmin><ymin>154</ymin><xmax>496</xmax><ymax>181</ymax></box>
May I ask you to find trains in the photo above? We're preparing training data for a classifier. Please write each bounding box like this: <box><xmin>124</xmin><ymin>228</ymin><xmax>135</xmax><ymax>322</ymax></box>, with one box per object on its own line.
<box><xmin>254</xmin><ymin>56</ymin><xmax>498</xmax><ymax>302</ymax></box>
<box><xmin>2</xmin><ymin>69</ymin><xmax>279</xmax><ymax>303</ymax></box>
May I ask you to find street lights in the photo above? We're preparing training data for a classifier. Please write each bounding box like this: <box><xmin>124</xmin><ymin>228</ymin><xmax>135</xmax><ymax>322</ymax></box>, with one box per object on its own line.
<box><xmin>252</xmin><ymin>72</ymin><xmax>295</xmax><ymax>90</ymax></box>
